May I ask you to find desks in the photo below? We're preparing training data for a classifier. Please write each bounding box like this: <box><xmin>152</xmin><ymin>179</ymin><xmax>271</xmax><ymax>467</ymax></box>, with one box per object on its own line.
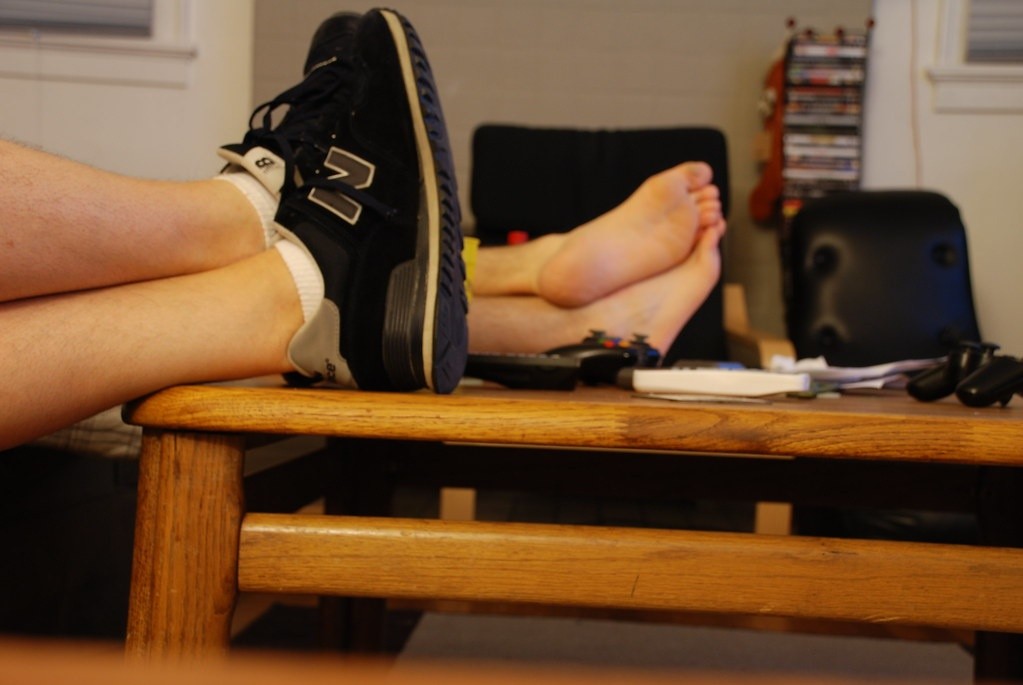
<box><xmin>120</xmin><ymin>394</ymin><xmax>1023</xmax><ymax>684</ymax></box>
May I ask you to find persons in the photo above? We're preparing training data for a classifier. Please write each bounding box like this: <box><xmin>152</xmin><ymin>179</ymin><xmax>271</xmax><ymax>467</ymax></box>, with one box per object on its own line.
<box><xmin>462</xmin><ymin>161</ymin><xmax>728</xmax><ymax>365</ymax></box>
<box><xmin>0</xmin><ymin>7</ymin><xmax>470</xmax><ymax>455</ymax></box>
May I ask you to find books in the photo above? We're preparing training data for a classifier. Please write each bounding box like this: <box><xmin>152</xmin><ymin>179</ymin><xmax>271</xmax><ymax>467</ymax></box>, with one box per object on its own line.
<box><xmin>782</xmin><ymin>35</ymin><xmax>869</xmax><ymax>199</ymax></box>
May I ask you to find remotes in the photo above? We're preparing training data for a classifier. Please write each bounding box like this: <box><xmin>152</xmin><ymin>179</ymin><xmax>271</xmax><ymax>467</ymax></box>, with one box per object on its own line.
<box><xmin>463</xmin><ymin>351</ymin><xmax>581</xmax><ymax>394</ymax></box>
<box><xmin>632</xmin><ymin>368</ymin><xmax>811</xmax><ymax>397</ymax></box>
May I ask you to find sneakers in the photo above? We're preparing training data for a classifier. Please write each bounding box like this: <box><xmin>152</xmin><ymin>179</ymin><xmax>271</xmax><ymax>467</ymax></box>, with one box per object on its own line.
<box><xmin>219</xmin><ymin>12</ymin><xmax>363</xmax><ymax>199</ymax></box>
<box><xmin>275</xmin><ymin>8</ymin><xmax>469</xmax><ymax>393</ymax></box>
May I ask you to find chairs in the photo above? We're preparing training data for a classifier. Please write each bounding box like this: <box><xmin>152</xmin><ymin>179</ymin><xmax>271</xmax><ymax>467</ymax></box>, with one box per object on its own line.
<box><xmin>775</xmin><ymin>196</ymin><xmax>1023</xmax><ymax>685</ymax></box>
<box><xmin>440</xmin><ymin>122</ymin><xmax>797</xmax><ymax>534</ymax></box>
